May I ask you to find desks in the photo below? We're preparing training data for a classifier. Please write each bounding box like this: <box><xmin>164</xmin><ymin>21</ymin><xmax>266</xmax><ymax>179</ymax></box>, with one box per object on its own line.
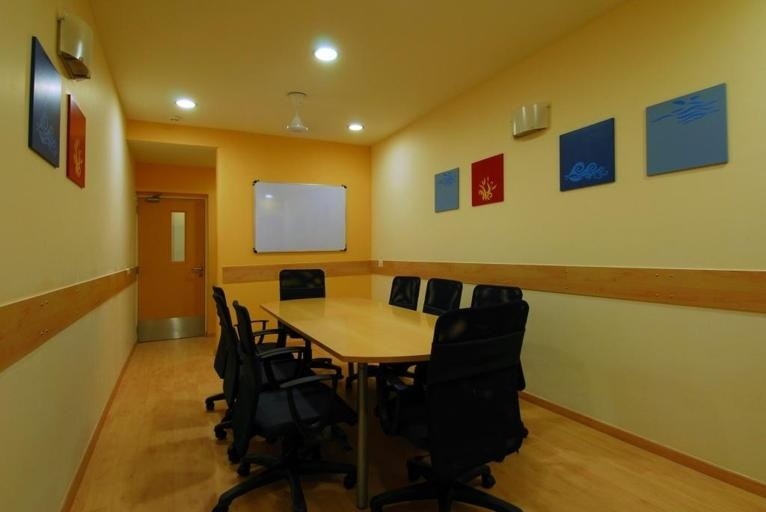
<box><xmin>259</xmin><ymin>298</ymin><xmax>460</xmax><ymax>509</ymax></box>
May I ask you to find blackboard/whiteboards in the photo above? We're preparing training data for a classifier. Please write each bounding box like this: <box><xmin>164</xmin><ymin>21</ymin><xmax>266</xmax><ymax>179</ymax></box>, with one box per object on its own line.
<box><xmin>253</xmin><ymin>179</ymin><xmax>347</xmax><ymax>254</ymax></box>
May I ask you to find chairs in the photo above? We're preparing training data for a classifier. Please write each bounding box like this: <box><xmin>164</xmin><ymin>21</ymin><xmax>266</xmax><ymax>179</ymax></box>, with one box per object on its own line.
<box><xmin>363</xmin><ymin>299</ymin><xmax>531</xmax><ymax>512</ymax></box>
<box><xmin>346</xmin><ymin>276</ymin><xmax>422</xmax><ymax>394</ymax></box>
<box><xmin>278</xmin><ymin>266</ymin><xmax>344</xmax><ymax>379</ymax></box>
<box><xmin>206</xmin><ymin>282</ymin><xmax>347</xmax><ymax>510</ymax></box>
<box><xmin>383</xmin><ymin>277</ymin><xmax>464</xmax><ymax>427</ymax></box>
<box><xmin>469</xmin><ymin>283</ymin><xmax>523</xmax><ymax>311</ymax></box>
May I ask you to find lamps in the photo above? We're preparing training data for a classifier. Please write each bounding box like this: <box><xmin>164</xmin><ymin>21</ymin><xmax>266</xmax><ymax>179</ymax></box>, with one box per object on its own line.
<box><xmin>285</xmin><ymin>91</ymin><xmax>309</xmax><ymax>133</ymax></box>
